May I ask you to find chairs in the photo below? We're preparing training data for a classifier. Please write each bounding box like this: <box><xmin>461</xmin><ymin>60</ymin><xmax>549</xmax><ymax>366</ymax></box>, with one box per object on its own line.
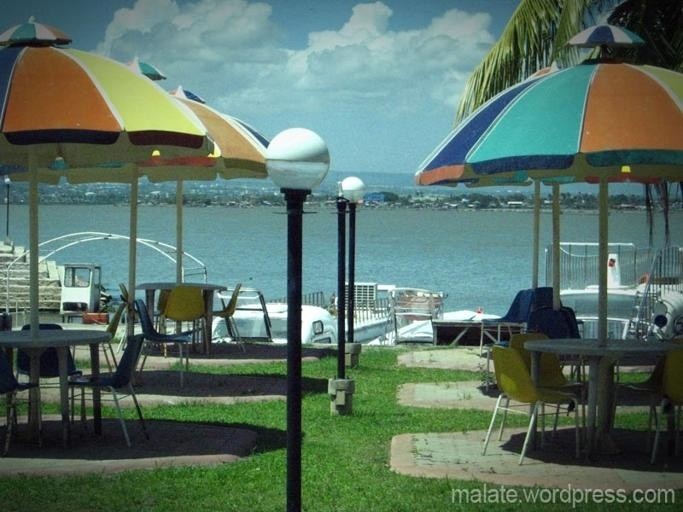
<box><xmin>477</xmin><ymin>286</ymin><xmax>683</xmax><ymax>472</ymax></box>
<box><xmin>0</xmin><ymin>280</ymin><xmax>274</xmax><ymax>454</ymax></box>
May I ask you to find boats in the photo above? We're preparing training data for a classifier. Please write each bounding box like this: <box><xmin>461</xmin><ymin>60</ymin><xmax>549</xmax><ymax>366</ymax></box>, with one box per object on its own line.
<box><xmin>211</xmin><ymin>281</ymin><xmax>450</xmax><ymax>346</ymax></box>
<box><xmin>541</xmin><ymin>241</ymin><xmax>683</xmax><ymax>339</ymax></box>
<box><xmin>0</xmin><ymin>231</ymin><xmax>211</xmax><ymax>342</ymax></box>
<box><xmin>365</xmin><ymin>307</ymin><xmax>504</xmax><ymax>348</ymax></box>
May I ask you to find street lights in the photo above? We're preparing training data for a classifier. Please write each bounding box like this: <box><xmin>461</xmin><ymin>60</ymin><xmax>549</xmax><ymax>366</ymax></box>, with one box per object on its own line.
<box><xmin>327</xmin><ymin>180</ymin><xmax>354</xmax><ymax>417</ymax></box>
<box><xmin>3</xmin><ymin>176</ymin><xmax>11</xmax><ymax>245</ymax></box>
<box><xmin>264</xmin><ymin>128</ymin><xmax>330</xmax><ymax>512</ymax></box>
<box><xmin>339</xmin><ymin>175</ymin><xmax>364</xmax><ymax>369</ymax></box>
